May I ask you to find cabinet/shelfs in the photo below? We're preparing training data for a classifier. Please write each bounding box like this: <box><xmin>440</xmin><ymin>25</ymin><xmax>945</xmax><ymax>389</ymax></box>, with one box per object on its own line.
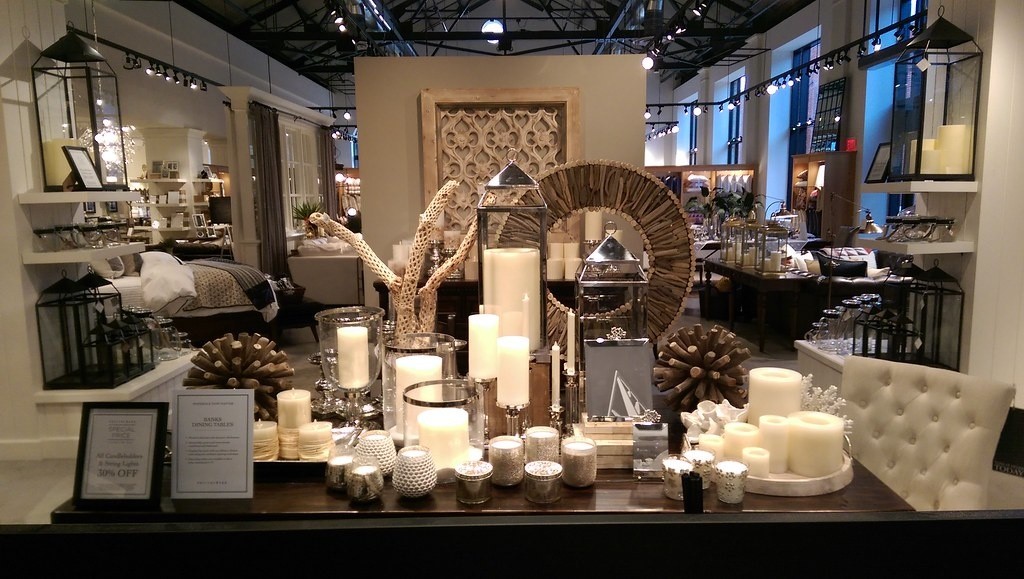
<box><xmin>791</xmin><ymin>150</ymin><xmax>858</xmax><ymax>248</ymax></box>
<box><xmin>795</xmin><ymin>181</ymin><xmax>979</xmax><ymax>407</ymax></box>
<box><xmin>130</xmin><ymin>178</ymin><xmax>224</xmax><ymax>232</ymax></box>
<box><xmin>645</xmin><ymin>163</ymin><xmax>759</xmax><ymax>224</ymax></box>
<box><xmin>17</xmin><ymin>189</ymin><xmax>208</xmax><ymax>437</ymax></box>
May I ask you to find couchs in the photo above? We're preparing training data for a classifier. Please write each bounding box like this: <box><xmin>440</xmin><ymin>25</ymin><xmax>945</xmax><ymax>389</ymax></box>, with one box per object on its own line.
<box><xmin>288</xmin><ymin>232</ymin><xmax>364</xmax><ymax>305</ymax></box>
<box><xmin>820</xmin><ymin>247</ymin><xmax>914</xmax><ymax>319</ymax></box>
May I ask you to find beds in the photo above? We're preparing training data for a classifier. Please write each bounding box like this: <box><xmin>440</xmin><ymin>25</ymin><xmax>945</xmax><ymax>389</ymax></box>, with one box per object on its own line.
<box><xmin>89</xmin><ymin>250</ymin><xmax>278</xmax><ymax>350</ymax></box>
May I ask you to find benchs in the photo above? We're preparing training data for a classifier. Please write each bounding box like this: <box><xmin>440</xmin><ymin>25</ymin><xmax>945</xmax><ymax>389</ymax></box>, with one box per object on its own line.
<box><xmin>841</xmin><ymin>353</ymin><xmax>1017</xmax><ymax>510</ymax></box>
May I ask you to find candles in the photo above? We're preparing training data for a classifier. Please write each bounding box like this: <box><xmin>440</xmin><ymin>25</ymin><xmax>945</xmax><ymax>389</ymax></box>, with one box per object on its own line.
<box><xmin>252</xmin><ymin>386</ymin><xmax>312</xmax><ymax>462</ymax></box>
<box><xmin>722</xmin><ymin>242</ymin><xmax>781</xmax><ymax>270</ymax></box>
<box><xmin>336</xmin><ymin>326</ymin><xmax>371</xmax><ymax>389</ymax></box>
<box><xmin>744</xmin><ymin>366</ymin><xmax>847</xmax><ymax>478</ymax></box>
<box><xmin>495</xmin><ymin>336</ymin><xmax>531</xmax><ymax>405</ymax></box>
<box><xmin>487</xmin><ymin>425</ymin><xmax>597</xmax><ymax>489</ymax></box>
<box><xmin>723</xmin><ymin>422</ymin><xmax>758</xmax><ymax>463</ymax></box>
<box><xmin>298</xmin><ymin>419</ymin><xmax>437</xmax><ymax>504</ymax></box>
<box><xmin>552</xmin><ymin>341</ymin><xmax>560</xmax><ymax>407</ymax></box>
<box><xmin>661</xmin><ymin>455</ymin><xmax>696</xmax><ymax>500</ymax></box>
<box><xmin>697</xmin><ymin>433</ymin><xmax>724</xmax><ymax>464</ymax></box>
<box><xmin>468</xmin><ymin>314</ymin><xmax>499</xmax><ymax>379</ymax></box>
<box><xmin>715</xmin><ymin>461</ymin><xmax>749</xmax><ymax>504</ymax></box>
<box><xmin>417</xmin><ymin>407</ymin><xmax>470</xmax><ymax>469</ymax></box>
<box><xmin>388</xmin><ymin>210</ymin><xmax>624</xmax><ymax>351</ymax></box>
<box><xmin>682</xmin><ymin>449</ymin><xmax>714</xmax><ymax>489</ymax></box>
<box><xmin>395</xmin><ymin>355</ymin><xmax>443</xmax><ymax>436</ymax></box>
<box><xmin>909</xmin><ymin>123</ymin><xmax>971</xmax><ymax>173</ymax></box>
<box><xmin>43</xmin><ymin>138</ymin><xmax>98</xmax><ymax>187</ymax></box>
<box><xmin>568</xmin><ymin>308</ymin><xmax>575</xmax><ymax>371</ymax></box>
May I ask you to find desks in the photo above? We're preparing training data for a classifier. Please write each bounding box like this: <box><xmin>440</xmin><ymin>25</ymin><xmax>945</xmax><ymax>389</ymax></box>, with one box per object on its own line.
<box><xmin>50</xmin><ymin>455</ymin><xmax>916</xmax><ymax>525</ymax></box>
<box><xmin>693</xmin><ymin>257</ymin><xmax>825</xmax><ymax>353</ymax></box>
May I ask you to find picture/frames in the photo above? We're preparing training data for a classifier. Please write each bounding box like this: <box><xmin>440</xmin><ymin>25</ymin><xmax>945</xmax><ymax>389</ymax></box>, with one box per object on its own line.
<box><xmin>864</xmin><ymin>142</ymin><xmax>891</xmax><ymax>184</ymax></box>
<box><xmin>106</xmin><ymin>201</ymin><xmax>119</xmax><ymax>213</ymax></box>
<box><xmin>84</xmin><ymin>202</ymin><xmax>96</xmax><ymax>214</ymax></box>
<box><xmin>72</xmin><ymin>402</ymin><xmax>169</xmax><ymax>509</ymax></box>
<box><xmin>61</xmin><ymin>145</ymin><xmax>105</xmax><ymax>191</ymax></box>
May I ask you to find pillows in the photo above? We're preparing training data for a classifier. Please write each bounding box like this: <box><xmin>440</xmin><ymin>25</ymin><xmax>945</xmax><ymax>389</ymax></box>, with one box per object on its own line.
<box><xmin>716</xmin><ymin>174</ymin><xmax>723</xmax><ymax>193</ymax></box>
<box><xmin>686</xmin><ymin>188</ymin><xmax>702</xmax><ymax>193</ymax></box>
<box><xmin>721</xmin><ymin>175</ymin><xmax>730</xmax><ymax>192</ymax></box>
<box><xmin>737</xmin><ymin>174</ymin><xmax>744</xmax><ymax>193</ymax></box>
<box><xmin>687</xmin><ymin>174</ymin><xmax>708</xmax><ymax>182</ymax></box>
<box><xmin>689</xmin><ymin>182</ymin><xmax>709</xmax><ymax>188</ymax></box>
<box><xmin>90</xmin><ymin>256</ymin><xmax>126</xmax><ymax>279</ymax></box>
<box><xmin>797</xmin><ymin>170</ymin><xmax>807</xmax><ymax>180</ymax></box>
<box><xmin>795</xmin><ymin>180</ymin><xmax>808</xmax><ymax>190</ymax></box>
<box><xmin>729</xmin><ymin>174</ymin><xmax>737</xmax><ymax>192</ymax></box>
<box><xmin>119</xmin><ymin>252</ymin><xmax>143</xmax><ymax>277</ymax></box>
<box><xmin>743</xmin><ymin>174</ymin><xmax>753</xmax><ymax>193</ymax></box>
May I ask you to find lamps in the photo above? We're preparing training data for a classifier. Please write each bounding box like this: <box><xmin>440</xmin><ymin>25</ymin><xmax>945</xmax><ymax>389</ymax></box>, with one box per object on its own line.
<box><xmin>684</xmin><ymin>106</ymin><xmax>688</xmax><ymax>115</ymax></box>
<box><xmin>481</xmin><ymin>19</ymin><xmax>503</xmax><ymax>44</ymax></box>
<box><xmin>330</xmin><ymin>110</ymin><xmax>358</xmax><ymax>144</ymax></box>
<box><xmin>666</xmin><ymin>20</ymin><xmax>688</xmax><ymax>41</ymax></box>
<box><xmin>655</xmin><ymin>36</ymin><xmax>664</xmax><ymax>53</ymax></box>
<box><xmin>826</xmin><ymin>193</ymin><xmax>885</xmax><ymax>310</ymax></box>
<box><xmin>332</xmin><ymin>12</ymin><xmax>356</xmax><ymax>48</ymax></box>
<box><xmin>652</xmin><ymin>41</ymin><xmax>660</xmax><ymax>57</ymax></box>
<box><xmin>703</xmin><ymin>105</ymin><xmax>709</xmax><ymax>113</ymax></box>
<box><xmin>693</xmin><ymin>106</ymin><xmax>702</xmax><ymax>116</ymax></box>
<box><xmin>693</xmin><ymin>2</ymin><xmax>710</xmax><ymax>17</ymax></box>
<box><xmin>123</xmin><ymin>52</ymin><xmax>208</xmax><ymax>92</ymax></box>
<box><xmin>642</xmin><ymin>57</ymin><xmax>654</xmax><ymax>70</ymax></box>
<box><xmin>719</xmin><ymin>20</ymin><xmax>921</xmax><ymax>146</ymax></box>
<box><xmin>657</xmin><ymin>107</ymin><xmax>662</xmax><ymax>115</ymax></box>
<box><xmin>644</xmin><ymin>106</ymin><xmax>652</xmax><ymax>119</ymax></box>
<box><xmin>325</xmin><ymin>3</ymin><xmax>337</xmax><ymax>15</ymax></box>
<box><xmin>645</xmin><ymin>123</ymin><xmax>679</xmax><ymax>143</ymax></box>
<box><xmin>765</xmin><ymin>200</ymin><xmax>799</xmax><ymax>219</ymax></box>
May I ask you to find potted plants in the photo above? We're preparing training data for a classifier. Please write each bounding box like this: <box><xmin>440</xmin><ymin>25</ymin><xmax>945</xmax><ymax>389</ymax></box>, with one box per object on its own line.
<box><xmin>291</xmin><ymin>200</ymin><xmax>324</xmax><ymax>240</ymax></box>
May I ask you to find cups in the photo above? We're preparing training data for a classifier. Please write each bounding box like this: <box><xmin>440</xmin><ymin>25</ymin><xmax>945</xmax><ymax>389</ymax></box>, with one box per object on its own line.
<box><xmin>402</xmin><ymin>380</ymin><xmax>485</xmax><ymax>483</ymax></box>
<box><xmin>317</xmin><ymin>306</ymin><xmax>384</xmax><ymax>417</ymax></box>
<box><xmin>380</xmin><ymin>331</ymin><xmax>457</xmax><ymax>446</ymax></box>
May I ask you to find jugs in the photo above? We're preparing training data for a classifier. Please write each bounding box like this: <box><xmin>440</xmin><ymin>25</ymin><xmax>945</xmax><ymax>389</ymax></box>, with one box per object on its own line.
<box><xmin>116</xmin><ymin>307</ymin><xmax>180</xmax><ymax>361</ymax></box>
